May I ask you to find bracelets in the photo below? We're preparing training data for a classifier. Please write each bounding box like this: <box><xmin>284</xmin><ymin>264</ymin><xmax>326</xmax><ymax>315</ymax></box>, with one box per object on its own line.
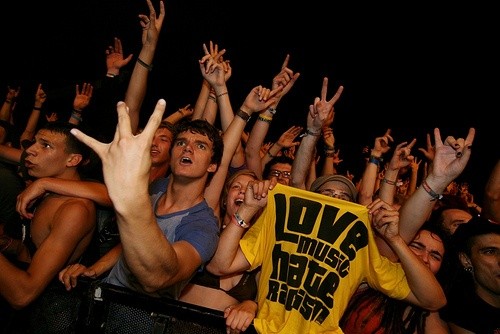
<box><xmin>383</xmin><ymin>178</ymin><xmax>396</xmax><ymax>185</ymax></box>
<box><xmin>422</xmin><ymin>176</ymin><xmax>444</xmax><ymax>201</ymax></box>
<box><xmin>106</xmin><ymin>73</ymin><xmax>116</xmax><ymax>78</ymax></box>
<box><xmin>4</xmin><ymin>100</ymin><xmax>12</xmax><ymax>103</ymax></box>
<box><xmin>257</xmin><ymin>113</ymin><xmax>272</xmax><ymax>123</ymax></box>
<box><xmin>266</xmin><ymin>106</ymin><xmax>276</xmax><ymax>114</ymax></box>
<box><xmin>305</xmin><ymin>129</ymin><xmax>322</xmax><ymax>136</ymax></box>
<box><xmin>70</xmin><ymin>108</ymin><xmax>82</xmax><ymax>123</ymax></box>
<box><xmin>208</xmin><ymin>89</ymin><xmax>228</xmax><ymax>103</ymax></box>
<box><xmin>267</xmin><ymin>148</ymin><xmax>277</xmax><ymax>159</ymax></box>
<box><xmin>32</xmin><ymin>107</ymin><xmax>42</xmax><ymax>110</ymax></box>
<box><xmin>323</xmin><ymin>149</ymin><xmax>335</xmax><ymax>157</ymax></box>
<box><xmin>368</xmin><ymin>155</ymin><xmax>379</xmax><ymax>165</ymax></box>
<box><xmin>138</xmin><ymin>57</ymin><xmax>154</xmax><ymax>71</ymax></box>
<box><xmin>231</xmin><ymin>211</ymin><xmax>249</xmax><ymax>228</ymax></box>
<box><xmin>178</xmin><ymin>109</ymin><xmax>184</xmax><ymax>116</ymax></box>
<box><xmin>236</xmin><ymin>109</ymin><xmax>251</xmax><ymax>122</ymax></box>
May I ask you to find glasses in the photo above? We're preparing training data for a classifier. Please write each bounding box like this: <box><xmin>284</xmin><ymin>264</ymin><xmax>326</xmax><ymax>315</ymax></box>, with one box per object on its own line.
<box><xmin>269</xmin><ymin>170</ymin><xmax>291</xmax><ymax>177</ymax></box>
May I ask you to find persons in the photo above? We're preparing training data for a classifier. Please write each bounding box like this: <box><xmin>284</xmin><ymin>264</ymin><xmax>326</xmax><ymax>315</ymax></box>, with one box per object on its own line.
<box><xmin>206</xmin><ymin>177</ymin><xmax>447</xmax><ymax>334</ymax></box>
<box><xmin>0</xmin><ymin>122</ymin><xmax>99</xmax><ymax>311</ymax></box>
<box><xmin>0</xmin><ymin>0</ymin><xmax>250</xmax><ymax>281</ymax></box>
<box><xmin>177</xmin><ymin>85</ymin><xmax>284</xmax><ymax>334</ymax></box>
<box><xmin>58</xmin><ymin>98</ymin><xmax>225</xmax><ymax>302</ymax></box>
<box><xmin>244</xmin><ymin>53</ymin><xmax>500</xmax><ymax>334</ymax></box>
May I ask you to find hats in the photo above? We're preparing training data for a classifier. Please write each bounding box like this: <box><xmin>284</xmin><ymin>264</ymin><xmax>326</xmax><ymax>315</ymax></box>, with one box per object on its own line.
<box><xmin>309</xmin><ymin>175</ymin><xmax>357</xmax><ymax>201</ymax></box>
